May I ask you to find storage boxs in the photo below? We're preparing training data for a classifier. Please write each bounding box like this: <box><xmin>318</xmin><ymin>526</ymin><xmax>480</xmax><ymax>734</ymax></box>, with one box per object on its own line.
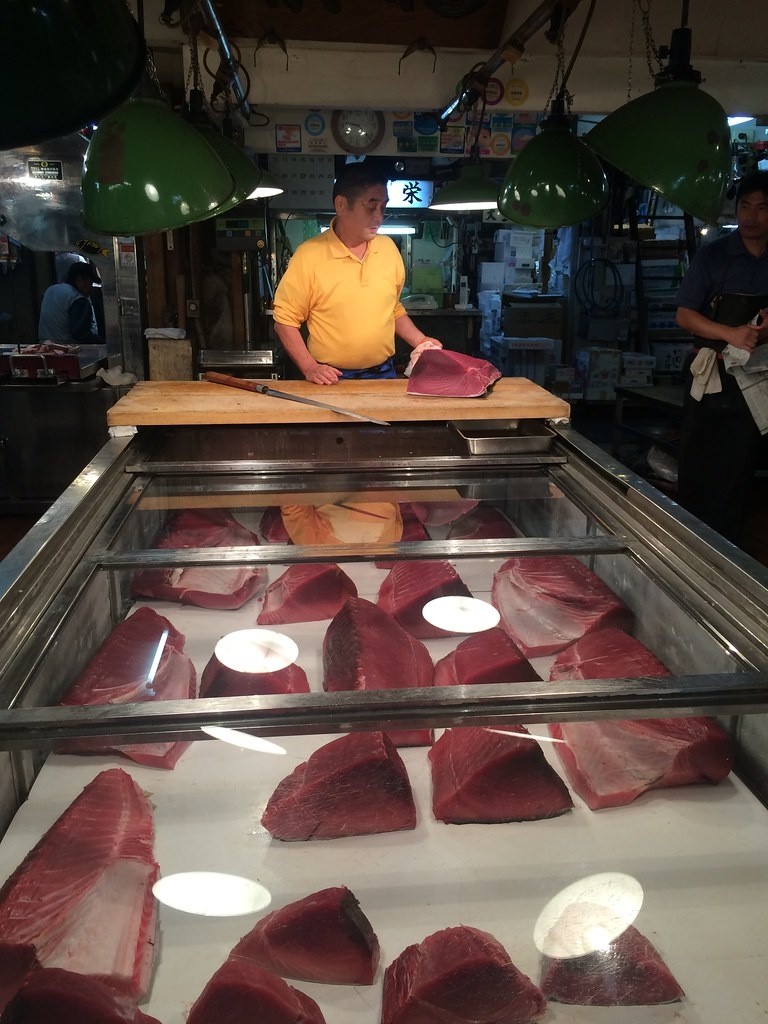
<box><xmin>477</xmin><ymin>229</ymin><xmax>694</xmax><ymax>401</ymax></box>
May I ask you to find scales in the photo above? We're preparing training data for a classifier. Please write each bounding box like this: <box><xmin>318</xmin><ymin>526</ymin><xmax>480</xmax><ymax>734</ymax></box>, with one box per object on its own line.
<box><xmin>197</xmin><ymin>196</ymin><xmax>283</xmax><ymax>366</ymax></box>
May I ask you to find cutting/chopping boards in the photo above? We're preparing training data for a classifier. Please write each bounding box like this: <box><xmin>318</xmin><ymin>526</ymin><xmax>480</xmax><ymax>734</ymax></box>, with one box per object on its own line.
<box><xmin>106</xmin><ymin>375</ymin><xmax>571</xmax><ymax>425</ymax></box>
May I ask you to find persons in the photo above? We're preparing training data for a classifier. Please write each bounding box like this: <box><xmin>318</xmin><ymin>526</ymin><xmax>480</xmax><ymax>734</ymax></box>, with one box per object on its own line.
<box><xmin>37</xmin><ymin>251</ymin><xmax>106</xmax><ymax>344</ymax></box>
<box><xmin>273</xmin><ymin>162</ymin><xmax>443</xmax><ymax>387</ymax></box>
<box><xmin>673</xmin><ymin>171</ymin><xmax>768</xmax><ymax>565</ymax></box>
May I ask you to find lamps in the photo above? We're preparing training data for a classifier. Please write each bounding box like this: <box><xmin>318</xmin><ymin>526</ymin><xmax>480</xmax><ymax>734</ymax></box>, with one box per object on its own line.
<box><xmin>319</xmin><ymin>218</ymin><xmax>419</xmax><ymax>235</ymax></box>
<box><xmin>498</xmin><ymin>0</ymin><xmax>614</xmax><ymax>228</ymax></box>
<box><xmin>578</xmin><ymin>0</ymin><xmax>733</xmax><ymax>227</ymax></box>
<box><xmin>426</xmin><ymin>87</ymin><xmax>502</xmax><ymax>211</ymax></box>
<box><xmin>0</xmin><ymin>0</ymin><xmax>286</xmax><ymax>237</ymax></box>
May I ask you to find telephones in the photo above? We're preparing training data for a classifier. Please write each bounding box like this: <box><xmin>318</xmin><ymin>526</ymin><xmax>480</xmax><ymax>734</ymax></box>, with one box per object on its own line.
<box><xmin>401</xmin><ymin>294</ymin><xmax>439</xmax><ymax>309</ymax></box>
<box><xmin>454</xmin><ymin>276</ymin><xmax>473</xmax><ymax>311</ymax></box>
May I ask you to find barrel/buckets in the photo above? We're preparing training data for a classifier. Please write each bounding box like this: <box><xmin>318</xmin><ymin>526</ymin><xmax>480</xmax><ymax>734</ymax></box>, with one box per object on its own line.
<box><xmin>148</xmin><ymin>337</ymin><xmax>192</xmax><ymax>381</ymax></box>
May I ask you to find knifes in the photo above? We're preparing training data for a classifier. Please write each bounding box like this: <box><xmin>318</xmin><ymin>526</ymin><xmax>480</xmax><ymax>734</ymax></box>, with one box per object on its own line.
<box><xmin>204</xmin><ymin>370</ymin><xmax>392</xmax><ymax>426</ymax></box>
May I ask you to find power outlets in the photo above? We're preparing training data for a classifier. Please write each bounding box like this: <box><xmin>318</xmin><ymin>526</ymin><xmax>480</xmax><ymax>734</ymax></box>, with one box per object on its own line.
<box><xmin>735</xmin><ymin>129</ymin><xmax>754</xmax><ymax>143</ymax></box>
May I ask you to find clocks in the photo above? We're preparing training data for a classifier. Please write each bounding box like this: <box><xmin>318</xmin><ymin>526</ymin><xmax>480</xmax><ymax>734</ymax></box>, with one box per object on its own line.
<box><xmin>331</xmin><ymin>109</ymin><xmax>385</xmax><ymax>154</ymax></box>
